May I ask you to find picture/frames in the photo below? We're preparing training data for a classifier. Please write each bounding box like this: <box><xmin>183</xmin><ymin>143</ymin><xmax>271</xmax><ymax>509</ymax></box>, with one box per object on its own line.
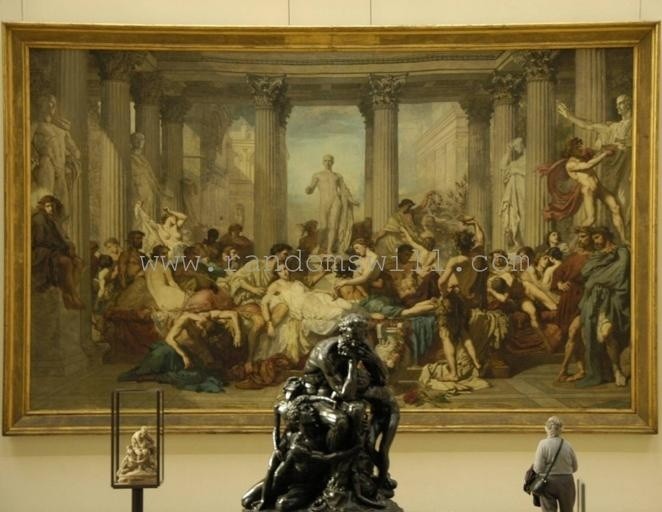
<box><xmin>0</xmin><ymin>20</ymin><xmax>661</xmax><ymax>439</ymax></box>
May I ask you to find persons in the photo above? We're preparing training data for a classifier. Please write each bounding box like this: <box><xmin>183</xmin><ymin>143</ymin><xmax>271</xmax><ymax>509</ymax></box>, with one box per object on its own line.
<box><xmin>531</xmin><ymin>416</ymin><xmax>579</xmax><ymax>512</ymax></box>
<box><xmin>30</xmin><ymin>93</ymin><xmax>633</xmax><ymax>388</ymax></box>
<box><xmin>304</xmin><ymin>313</ymin><xmax>408</xmax><ymax>489</ymax></box>
<box><xmin>240</xmin><ymin>394</ymin><xmax>364</xmax><ymax>512</ymax></box>
<box><xmin>342</xmin><ymin>397</ymin><xmax>388</xmax><ymax>509</ymax></box>
<box><xmin>117</xmin><ymin>425</ymin><xmax>156</xmax><ymax>482</ymax></box>
<box><xmin>255</xmin><ymin>375</ymin><xmax>338</xmax><ymax>512</ymax></box>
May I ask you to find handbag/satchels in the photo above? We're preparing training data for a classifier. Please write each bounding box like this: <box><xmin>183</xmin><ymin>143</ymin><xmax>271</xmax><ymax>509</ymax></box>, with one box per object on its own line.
<box><xmin>531</xmin><ymin>478</ymin><xmax>549</xmax><ymax>495</ymax></box>
